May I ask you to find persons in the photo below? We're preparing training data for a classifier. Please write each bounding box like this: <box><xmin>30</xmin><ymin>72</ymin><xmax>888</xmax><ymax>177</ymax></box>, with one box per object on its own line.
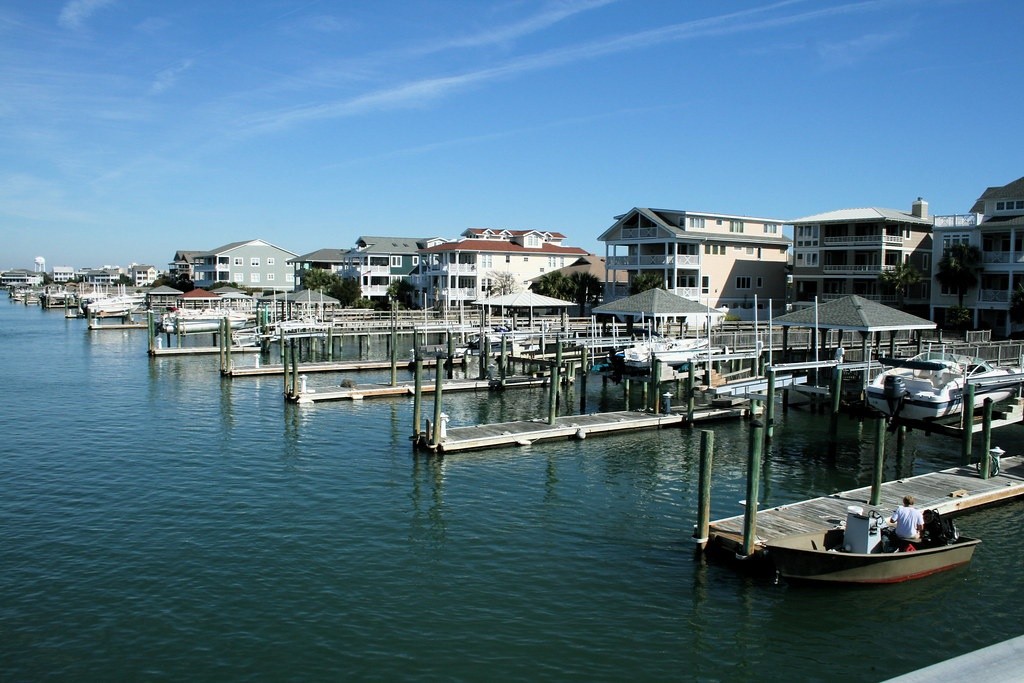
<box><xmin>890</xmin><ymin>496</ymin><xmax>925</xmax><ymax>539</ymax></box>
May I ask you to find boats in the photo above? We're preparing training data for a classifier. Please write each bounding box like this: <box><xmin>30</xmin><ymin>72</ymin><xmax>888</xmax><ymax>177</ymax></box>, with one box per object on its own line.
<box><xmin>764</xmin><ymin>521</ymin><xmax>982</xmax><ymax>584</ymax></box>
<box><xmin>49</xmin><ymin>290</ymin><xmax>140</xmax><ymax>318</ymax></box>
<box><xmin>466</xmin><ymin>328</ymin><xmax>534</xmax><ymax>349</ymax></box>
<box><xmin>867</xmin><ymin>349</ymin><xmax>1024</xmax><ymax>422</ymax></box>
<box><xmin>162</xmin><ymin>306</ymin><xmax>249</xmax><ymax>333</ymax></box>
<box><xmin>607</xmin><ymin>337</ymin><xmax>710</xmax><ymax>373</ymax></box>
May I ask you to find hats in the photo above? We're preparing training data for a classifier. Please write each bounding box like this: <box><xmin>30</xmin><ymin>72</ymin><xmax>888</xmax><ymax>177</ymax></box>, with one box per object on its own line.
<box><xmin>922</xmin><ymin>510</ymin><xmax>932</xmax><ymax>516</ymax></box>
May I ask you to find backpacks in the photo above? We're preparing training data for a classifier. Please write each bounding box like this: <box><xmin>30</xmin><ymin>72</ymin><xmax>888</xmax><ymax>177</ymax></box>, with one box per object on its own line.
<box><xmin>935</xmin><ymin>515</ymin><xmax>959</xmax><ymax>543</ymax></box>
